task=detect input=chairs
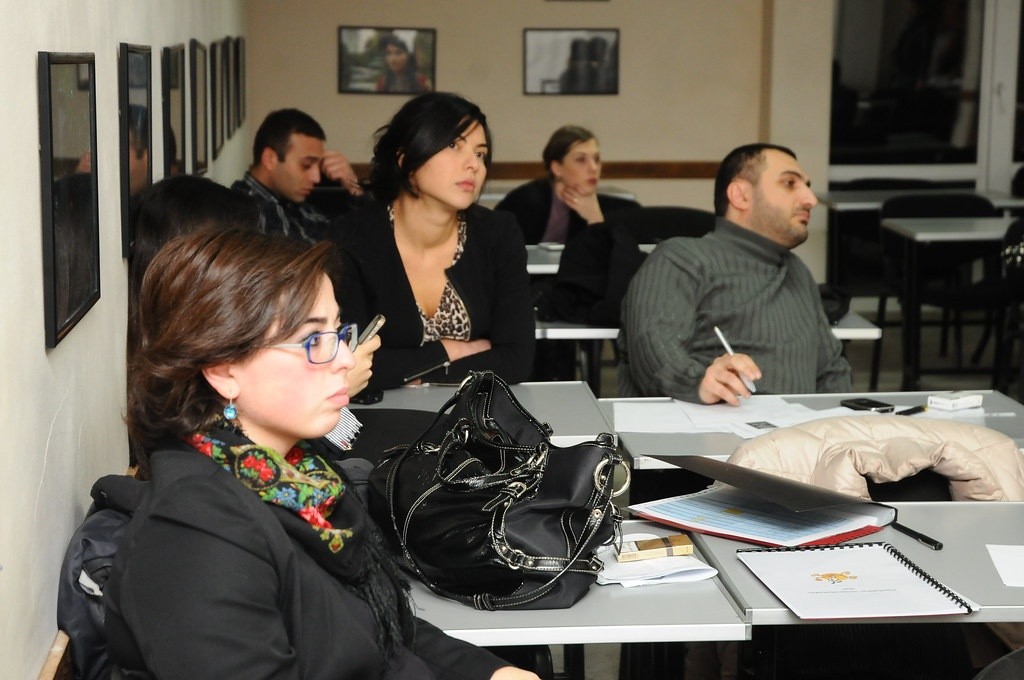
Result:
[868,191,1004,394]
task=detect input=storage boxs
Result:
[926,394,982,411]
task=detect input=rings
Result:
[573,198,577,202]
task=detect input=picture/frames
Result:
[522,28,619,96]
[38,35,247,350]
[337,25,437,95]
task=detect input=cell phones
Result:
[538,242,566,251]
[357,313,386,344]
[840,397,895,414]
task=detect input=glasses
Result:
[254,322,359,363]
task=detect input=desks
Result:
[356,191,1024,646]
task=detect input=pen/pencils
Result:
[890,521,943,552]
[713,325,758,395]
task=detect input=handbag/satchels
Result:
[367,370,631,610]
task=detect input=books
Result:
[740,541,980,619]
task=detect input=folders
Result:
[616,451,899,548]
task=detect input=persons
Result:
[494,124,617,380]
[129,105,177,192]
[230,109,364,242]
[50,172,97,322]
[129,175,250,332]
[106,226,541,680]
[319,91,536,388]
[613,143,853,507]
[375,35,432,92]
